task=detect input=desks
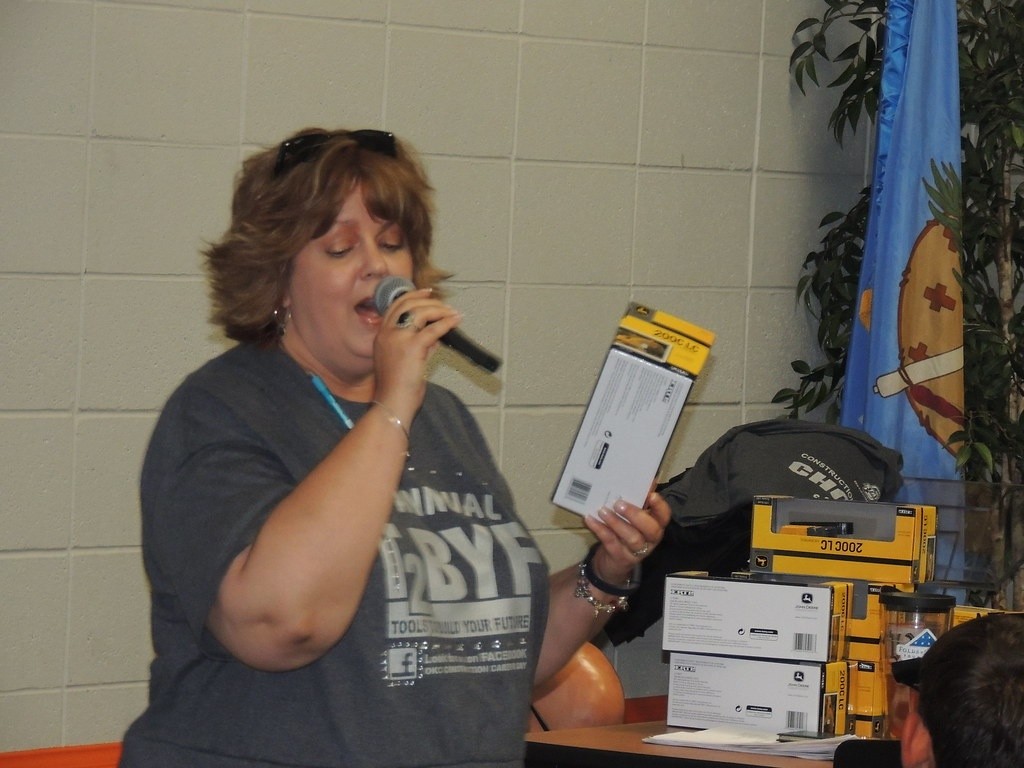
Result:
[524,720,832,766]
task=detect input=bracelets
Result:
[369,400,411,458]
[575,538,643,618]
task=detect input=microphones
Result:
[372,275,501,373]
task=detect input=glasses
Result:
[892,659,926,691]
[277,130,396,177]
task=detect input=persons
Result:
[900,614,1024,768]
[118,124,672,768]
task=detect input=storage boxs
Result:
[550,300,716,527]
[660,492,1024,739]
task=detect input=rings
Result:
[396,309,421,331]
[632,542,648,556]
